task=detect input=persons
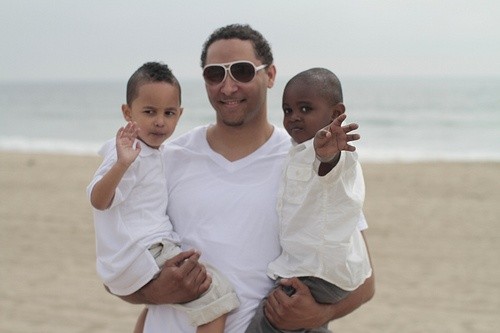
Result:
[89,61,240,331]
[132,23,331,332]
[260,68,369,333]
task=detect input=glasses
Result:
[202,60,267,85]
